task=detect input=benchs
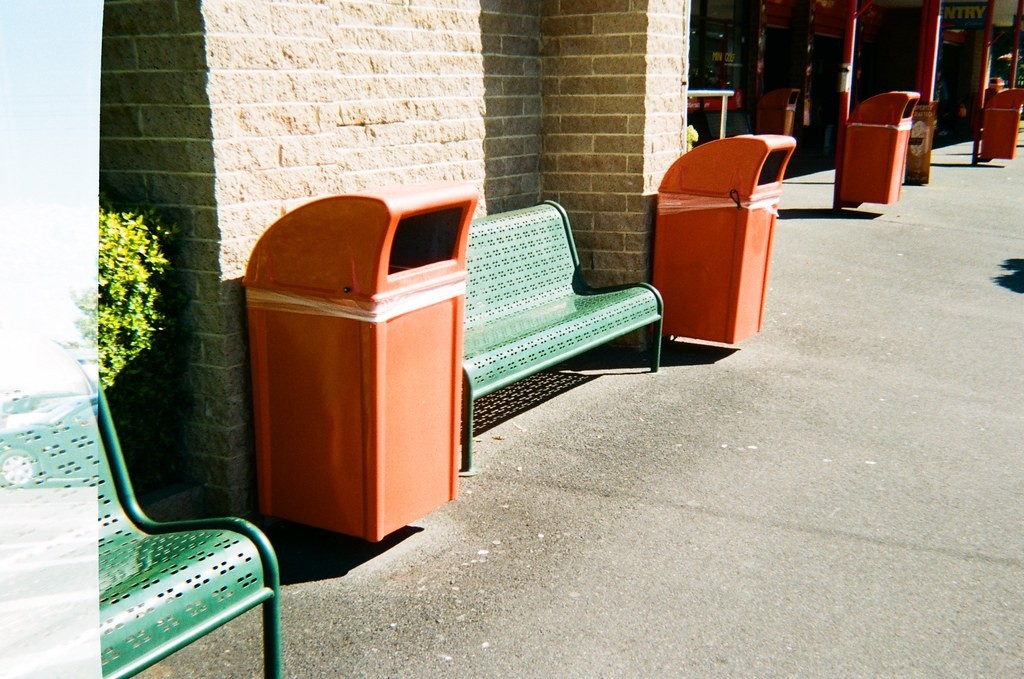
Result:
[0,383,285,679]
[460,199,665,478]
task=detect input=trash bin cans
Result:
[840,91,921,204]
[756,86,801,134]
[242,185,482,542]
[650,134,798,345]
[981,89,1024,160]
